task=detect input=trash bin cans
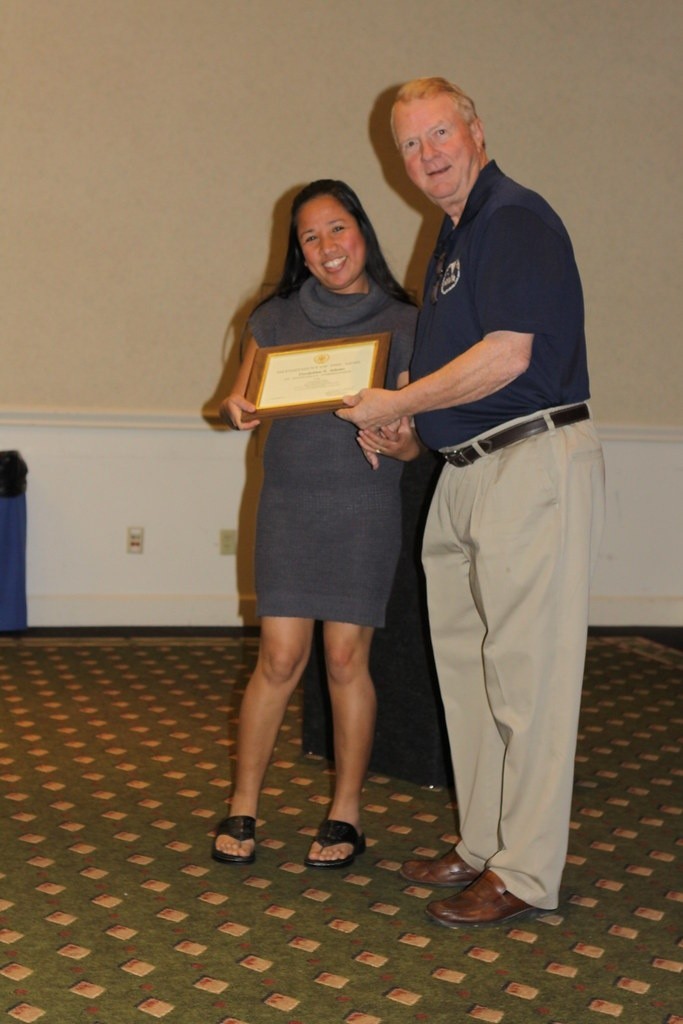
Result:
[0,449,30,634]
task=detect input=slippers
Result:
[304,820,368,868]
[210,817,255,863]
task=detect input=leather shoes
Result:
[399,844,478,887]
[426,864,554,931]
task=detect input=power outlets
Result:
[126,527,145,555]
[220,529,237,556]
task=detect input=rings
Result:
[376,449,381,454]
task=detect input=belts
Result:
[441,403,589,467]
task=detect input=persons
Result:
[209,179,419,870]
[336,78,608,930]
